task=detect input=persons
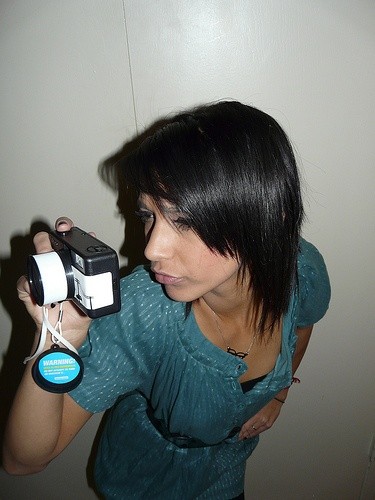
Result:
[2,100,333,499]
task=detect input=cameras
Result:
[28,226,121,319]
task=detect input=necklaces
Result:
[208,305,262,360]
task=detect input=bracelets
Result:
[274,397,286,404]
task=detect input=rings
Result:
[251,425,258,430]
